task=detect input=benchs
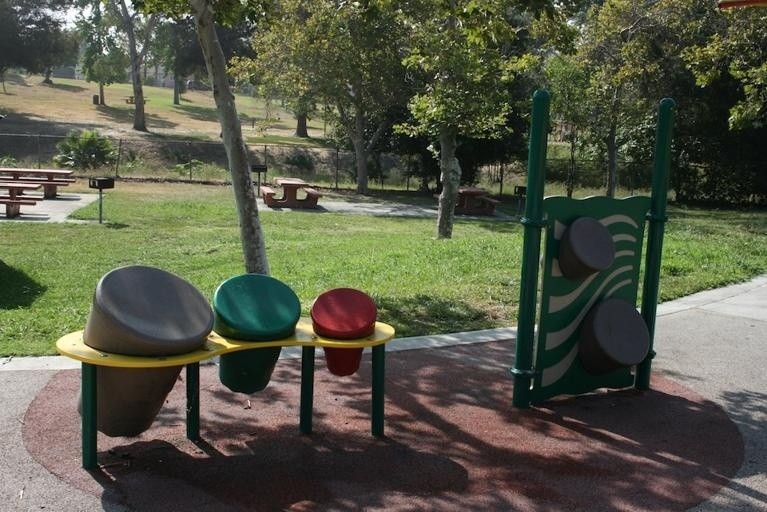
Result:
[477,196,501,216]
[0,176,76,217]
[258,185,276,208]
[302,186,321,208]
[432,192,441,201]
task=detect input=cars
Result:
[185,78,211,92]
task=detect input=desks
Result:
[273,176,308,208]
[454,186,487,215]
[0,168,74,189]
